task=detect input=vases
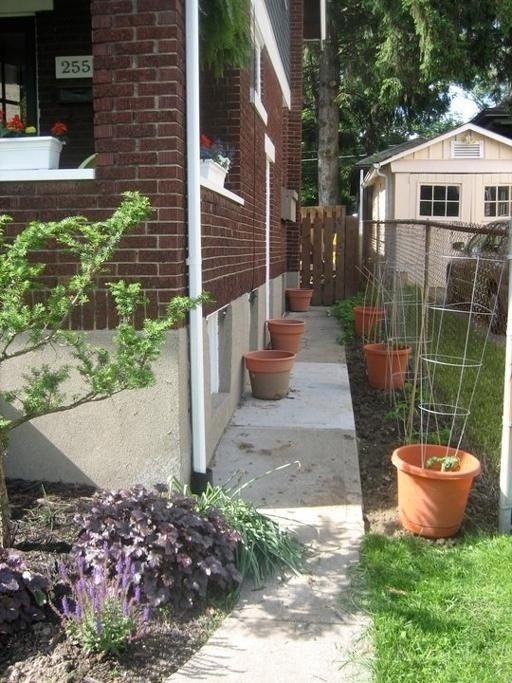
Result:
[0,136,63,169]
[200,159,229,188]
[244,350,296,400]
[268,319,305,354]
[286,288,314,312]
[353,306,387,337]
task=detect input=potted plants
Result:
[363,339,412,389]
[392,443,481,538]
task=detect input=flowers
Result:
[200,134,236,171]
[0,109,69,140]
